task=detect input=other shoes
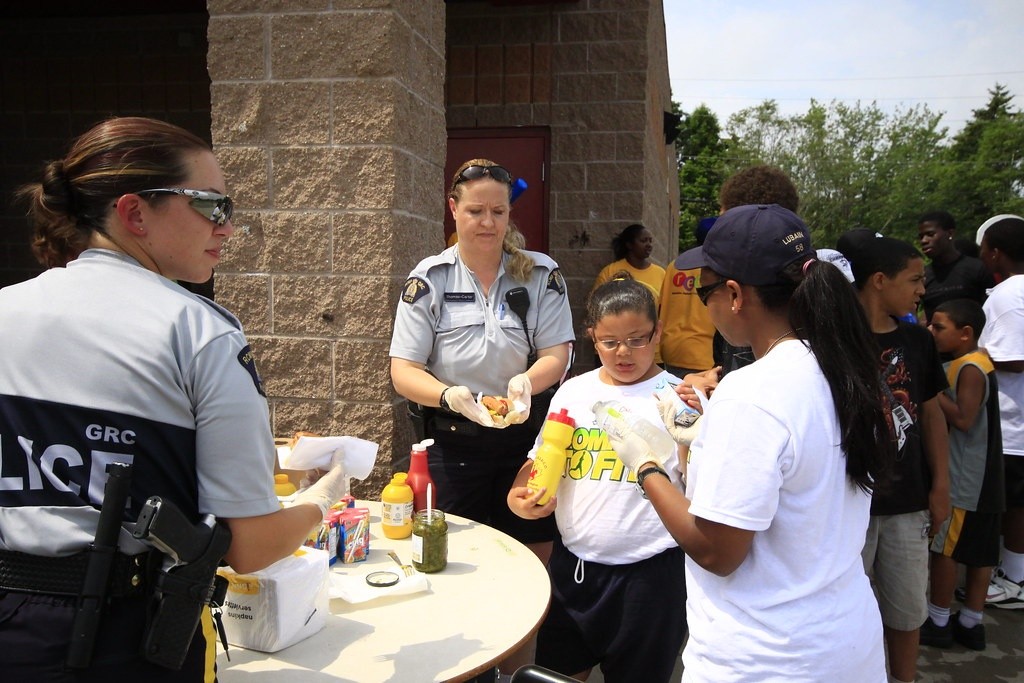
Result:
[919,614,955,649]
[949,610,987,651]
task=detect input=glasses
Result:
[449,164,513,194]
[592,321,657,350]
[695,276,731,308]
[111,187,234,227]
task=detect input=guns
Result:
[130,497,234,670]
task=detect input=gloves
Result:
[607,422,665,479]
[289,447,349,522]
[445,386,508,429]
[656,398,702,446]
[503,373,532,425]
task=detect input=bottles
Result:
[588,397,674,465]
[380,439,437,540]
[524,409,575,506]
[274,474,297,496]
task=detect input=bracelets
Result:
[635,465,671,501]
[439,387,457,415]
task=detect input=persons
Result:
[506,270,707,683]
[687,163,1024,682]
[390,159,575,683]
[608,202,891,683]
[0,118,348,683]
[593,224,667,371]
[661,215,720,380]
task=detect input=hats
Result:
[815,249,855,283]
[673,202,817,286]
[836,227,883,260]
[693,217,717,242]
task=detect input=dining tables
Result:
[210,496,552,683]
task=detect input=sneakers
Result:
[953,558,1024,611]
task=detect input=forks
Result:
[388,552,415,578]
